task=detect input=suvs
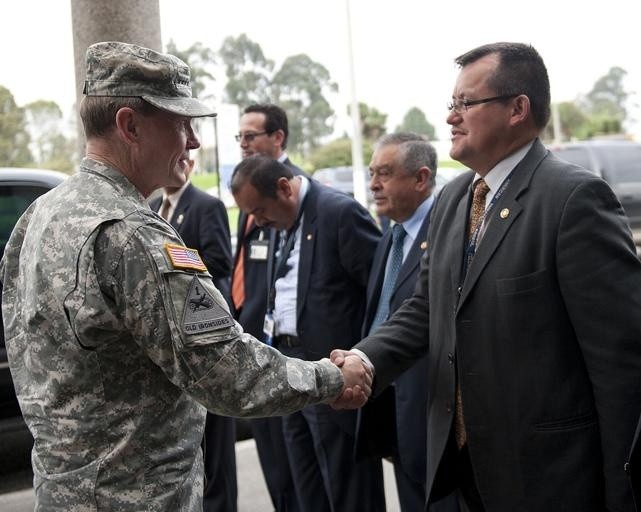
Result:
[544,139,641,248]
[312,165,372,205]
[0,168,251,458]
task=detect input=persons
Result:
[331,41,641,512]
[0,39,372,512]
[228,154,383,512]
[348,132,444,512]
[374,213,389,233]
[228,103,310,511]
[145,152,237,512]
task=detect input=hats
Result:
[84,41,218,117]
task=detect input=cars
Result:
[434,168,459,196]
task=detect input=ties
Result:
[231,214,255,309]
[161,199,170,220]
[368,224,407,336]
[465,178,491,270]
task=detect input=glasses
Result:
[235,131,269,141]
[447,94,517,113]
